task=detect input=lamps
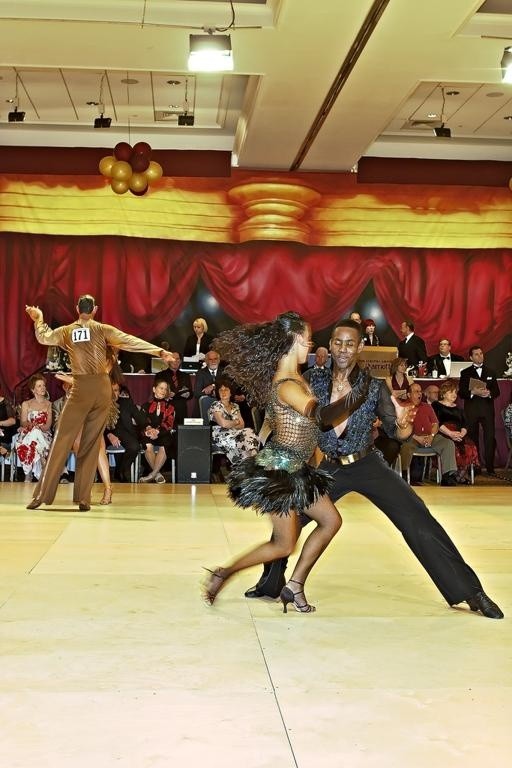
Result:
[186,26,234,74]
[432,124,452,138]
[94,117,112,128]
[501,46,512,84]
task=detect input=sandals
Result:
[24,471,167,484]
[100,487,112,505]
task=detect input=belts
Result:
[323,443,373,466]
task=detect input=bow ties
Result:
[442,356,450,360]
[476,365,482,369]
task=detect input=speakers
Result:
[176,425,211,484]
[433,127,451,136]
[94,117,113,127]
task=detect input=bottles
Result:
[431,359,439,378]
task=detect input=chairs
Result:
[0,454,7,482]
[501,409,512,473]
[104,450,135,484]
[390,453,402,478]
[251,406,263,436]
[199,395,229,483]
[406,448,443,487]
[134,402,176,484]
[428,453,476,485]
[14,400,25,482]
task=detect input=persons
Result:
[245,317,504,619]
[203,311,372,614]
[1,313,511,486]
[24,294,176,511]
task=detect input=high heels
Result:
[245,585,267,597]
[202,566,225,608]
[280,580,316,613]
[466,589,504,618]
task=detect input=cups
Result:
[418,364,426,379]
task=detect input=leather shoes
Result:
[78,500,90,510]
[26,498,52,508]
[442,468,497,486]
